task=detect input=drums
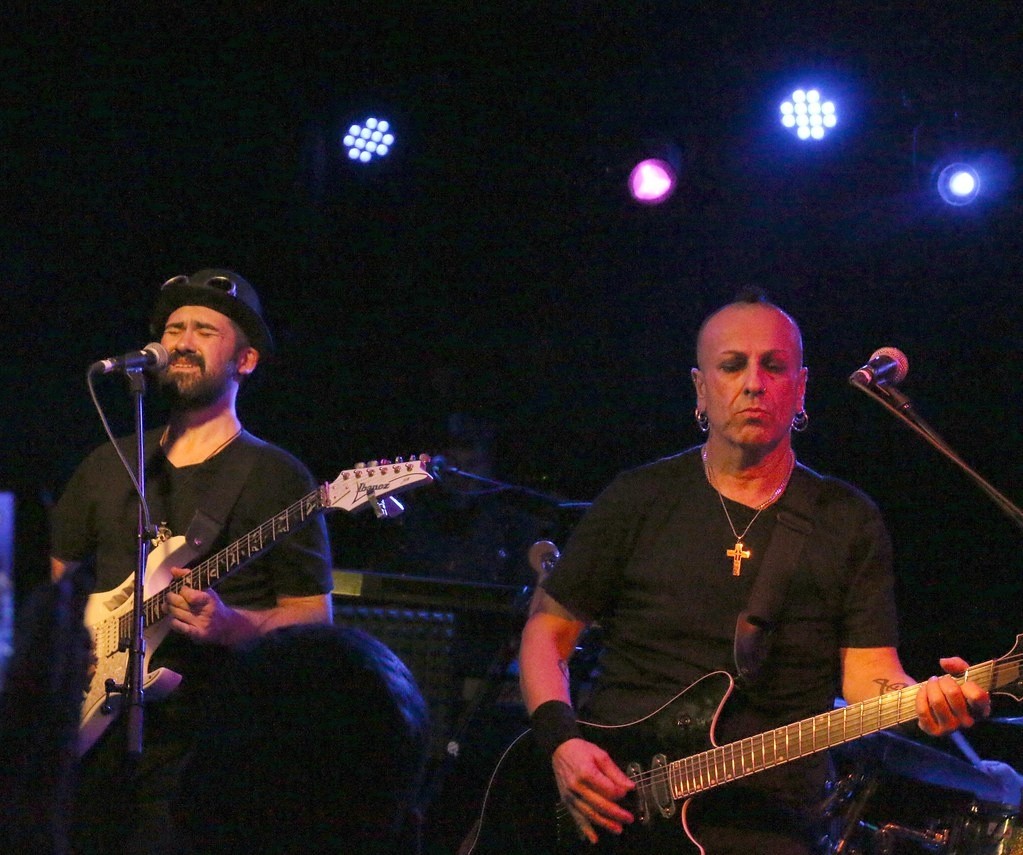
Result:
[924,799,1023,855]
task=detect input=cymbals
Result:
[989,716,1023,726]
[823,710,1004,797]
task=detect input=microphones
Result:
[529,541,560,574]
[848,346,908,387]
[90,342,168,375]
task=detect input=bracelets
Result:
[530,701,585,764]
[902,719,922,735]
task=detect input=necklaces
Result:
[162,428,241,462]
[706,448,794,576]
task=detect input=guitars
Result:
[464,634,1023,855]
[41,451,438,763]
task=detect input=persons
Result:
[518,299,992,855]
[182,623,426,855]
[49,268,333,855]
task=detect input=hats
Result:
[148,266,279,355]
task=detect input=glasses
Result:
[158,273,238,297]
